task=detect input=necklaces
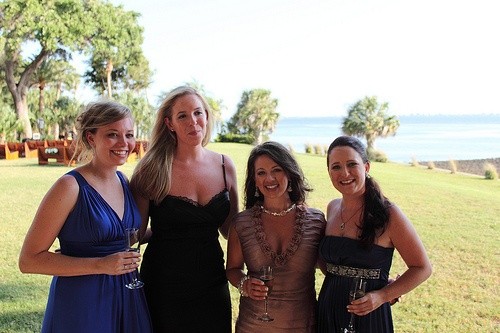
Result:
[261,203,297,216]
[340,203,364,230]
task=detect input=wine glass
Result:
[125,227,146,290]
[256,265,274,322]
[340,277,367,333]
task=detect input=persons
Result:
[313,135,433,333]
[18,100,153,333]
[226,141,398,333]
[128,83,239,333]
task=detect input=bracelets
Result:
[237,275,252,297]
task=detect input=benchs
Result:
[0,138,149,168]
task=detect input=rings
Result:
[123,265,126,269]
